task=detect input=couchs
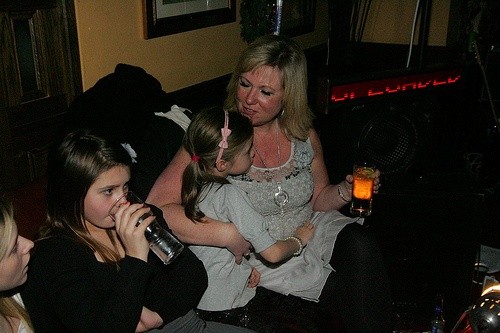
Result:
[1,160,391,333]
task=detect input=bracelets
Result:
[285,236,303,256]
[338,183,351,202]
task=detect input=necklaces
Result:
[252,118,290,207]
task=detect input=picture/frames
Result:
[141,0,236,40]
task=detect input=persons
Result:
[18,131,260,333]
[0,196,33,333]
[181,109,313,333]
[146,33,394,332]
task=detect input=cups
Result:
[108,192,184,264]
[350,162,377,216]
[473,264,488,284]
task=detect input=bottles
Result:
[428,306,445,333]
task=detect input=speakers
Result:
[353,102,435,200]
